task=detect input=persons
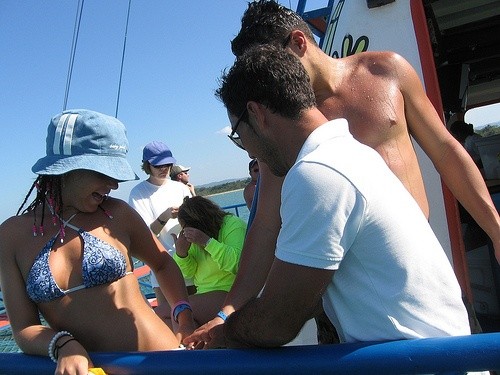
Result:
[129,0,500,349]
[0,110,192,375]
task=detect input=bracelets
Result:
[157,218,167,225]
[49,330,76,364]
[174,304,190,322]
[201,238,210,248]
[171,300,191,323]
[216,310,227,323]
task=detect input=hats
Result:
[143,141,176,166]
[31,109,141,180]
[171,165,190,179]
[249,159,256,171]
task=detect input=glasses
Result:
[228,108,247,150]
[251,169,259,172]
